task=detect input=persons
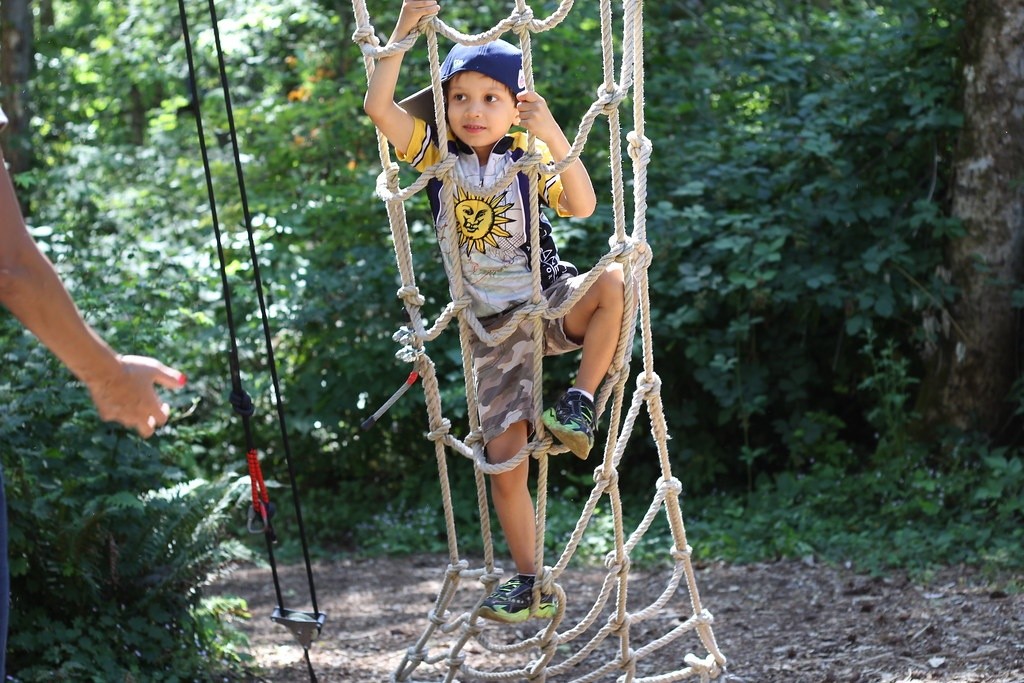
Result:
[0,105,187,437]
[363,0,638,624]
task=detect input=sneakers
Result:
[477,575,558,623]
[542,391,598,460]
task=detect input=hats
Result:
[399,39,525,138]
